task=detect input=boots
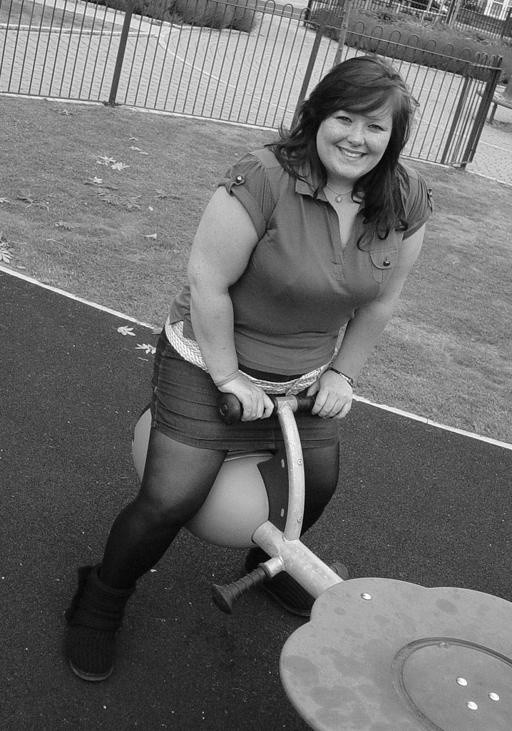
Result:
[67,564,137,681]
[247,549,315,617]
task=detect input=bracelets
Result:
[329,368,354,387]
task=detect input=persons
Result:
[63,56,436,683]
